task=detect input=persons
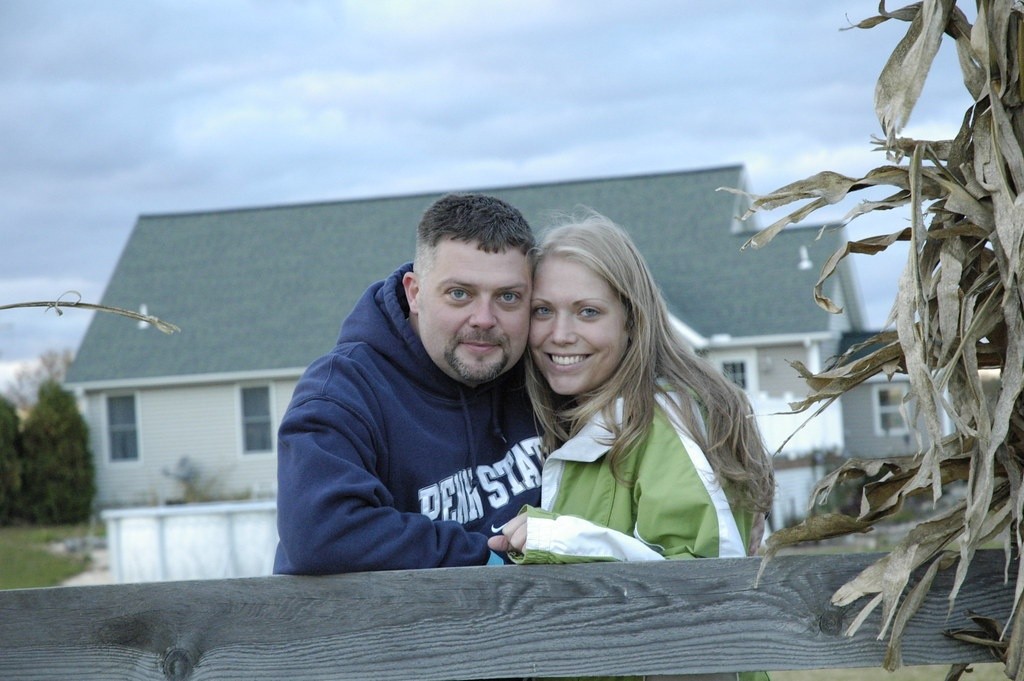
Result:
[273,194,548,577]
[487,209,776,566]
